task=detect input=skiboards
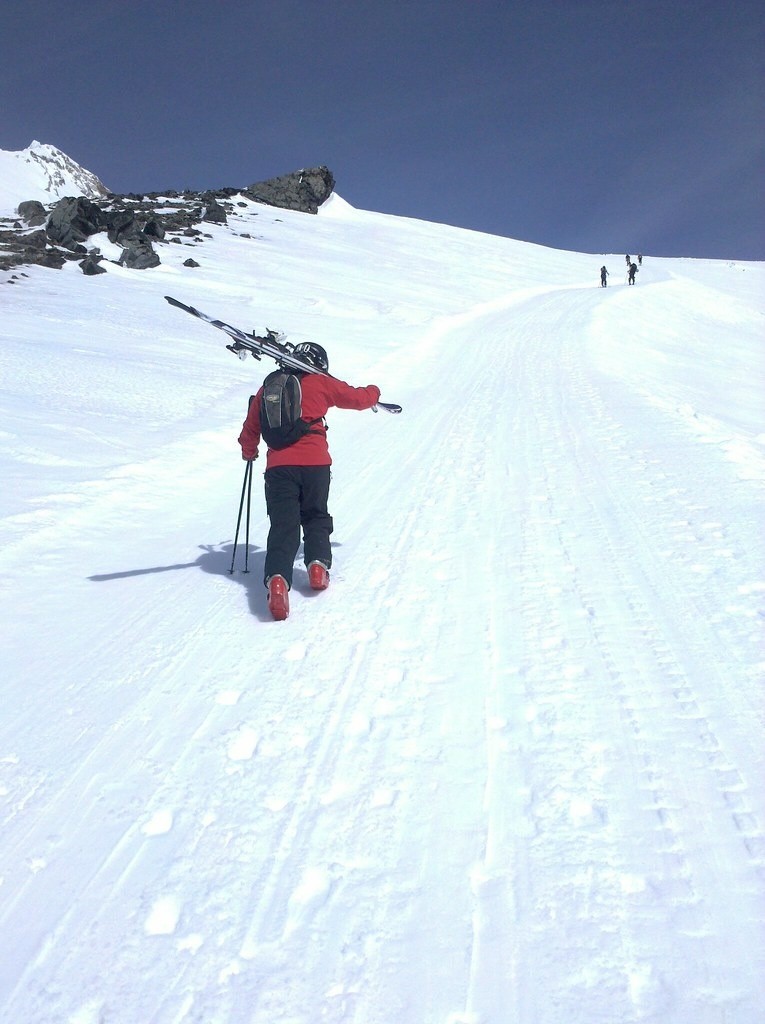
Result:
[166,295,405,415]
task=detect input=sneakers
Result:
[266,574,289,621]
[306,560,329,590]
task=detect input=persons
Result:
[239,342,380,622]
[600,253,643,287]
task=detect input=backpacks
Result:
[260,369,322,450]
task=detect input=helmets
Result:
[292,342,328,374]
